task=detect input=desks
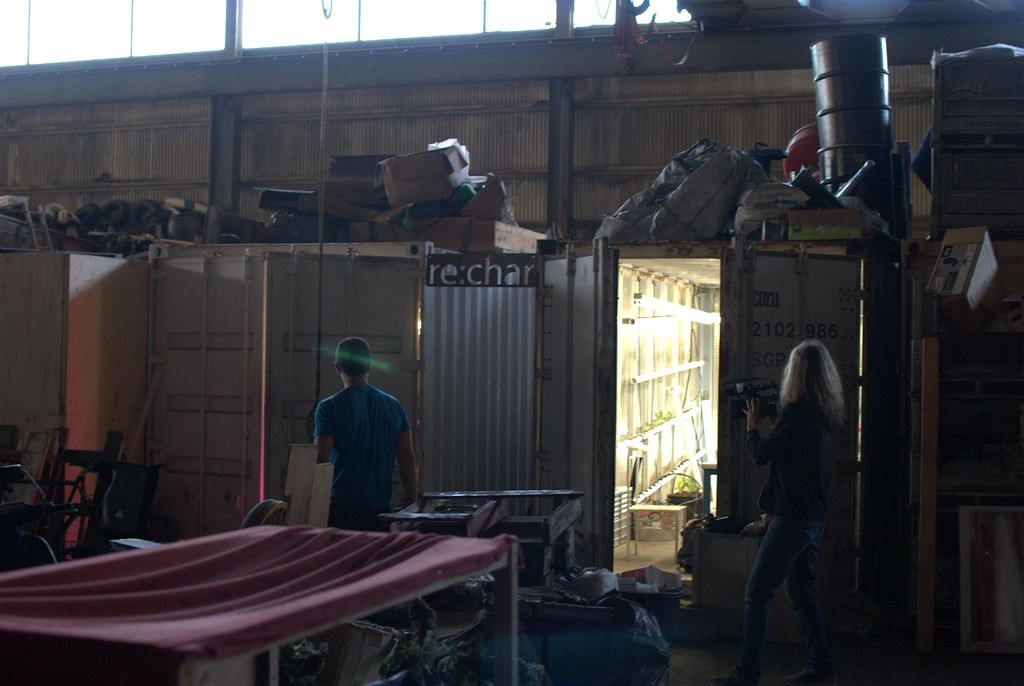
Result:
[626,504,687,561]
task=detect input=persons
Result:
[704,339,846,686]
[313,337,416,533]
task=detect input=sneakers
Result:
[706,672,759,686]
[783,664,838,686]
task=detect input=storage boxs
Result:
[255,137,547,254]
[921,226,1007,336]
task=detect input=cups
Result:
[622,565,656,584]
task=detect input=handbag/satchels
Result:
[756,479,775,512]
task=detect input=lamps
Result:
[631,359,705,385]
[620,292,721,331]
